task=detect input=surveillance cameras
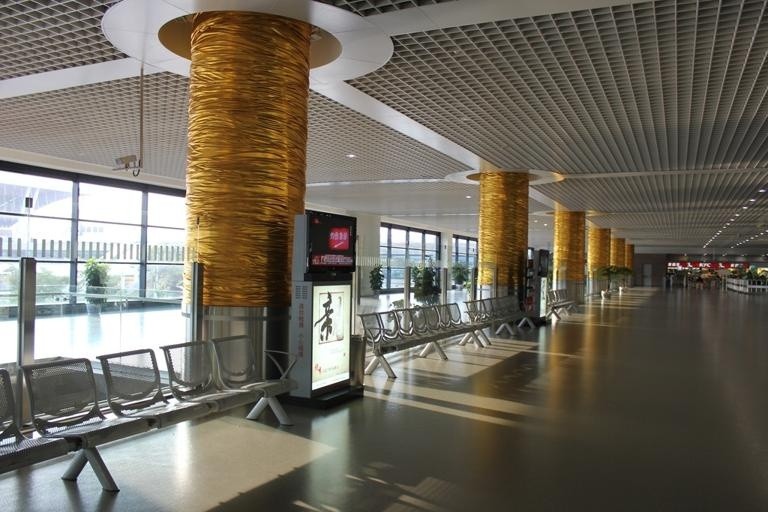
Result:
[115,155,137,166]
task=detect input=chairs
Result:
[355,287,580,379]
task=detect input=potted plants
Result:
[369,264,385,298]
[451,263,471,291]
[596,265,633,300]
[83,255,112,315]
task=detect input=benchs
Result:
[1,337,298,491]
